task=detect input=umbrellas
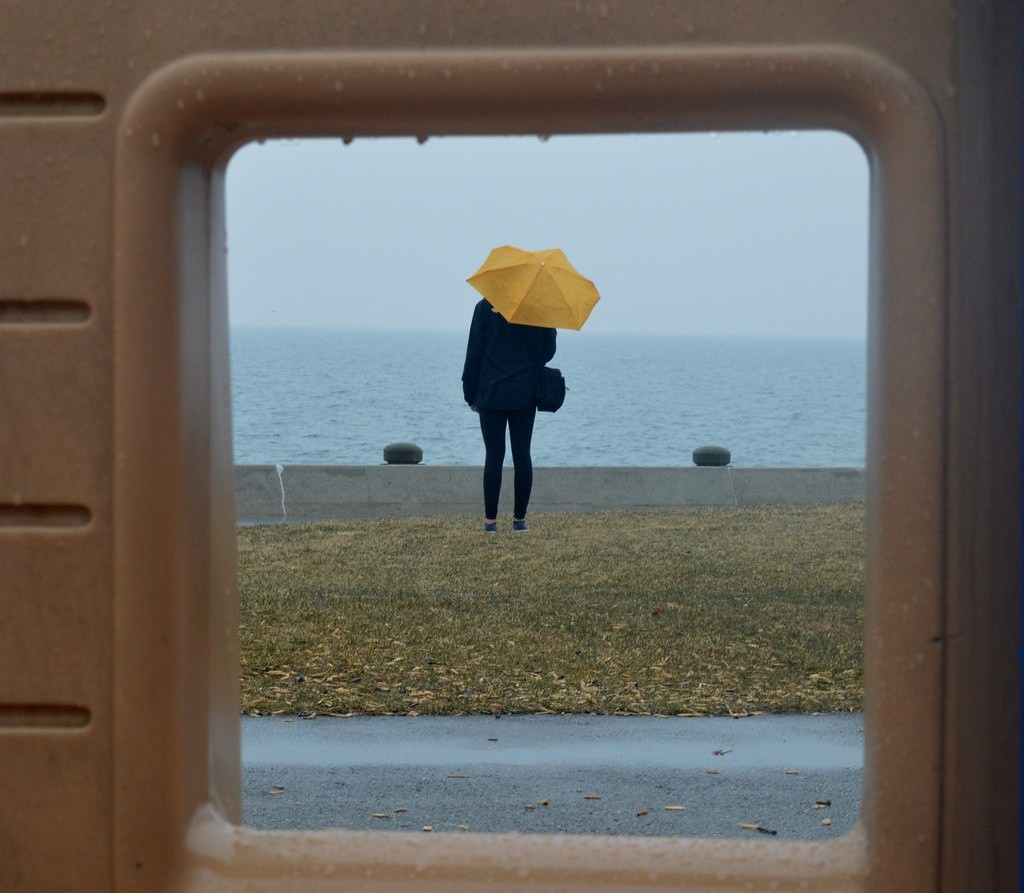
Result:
[466,245,600,330]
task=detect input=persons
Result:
[462,297,557,533]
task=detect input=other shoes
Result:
[485,524,497,533]
[513,521,529,531]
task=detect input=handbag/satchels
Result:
[525,367,567,413]
